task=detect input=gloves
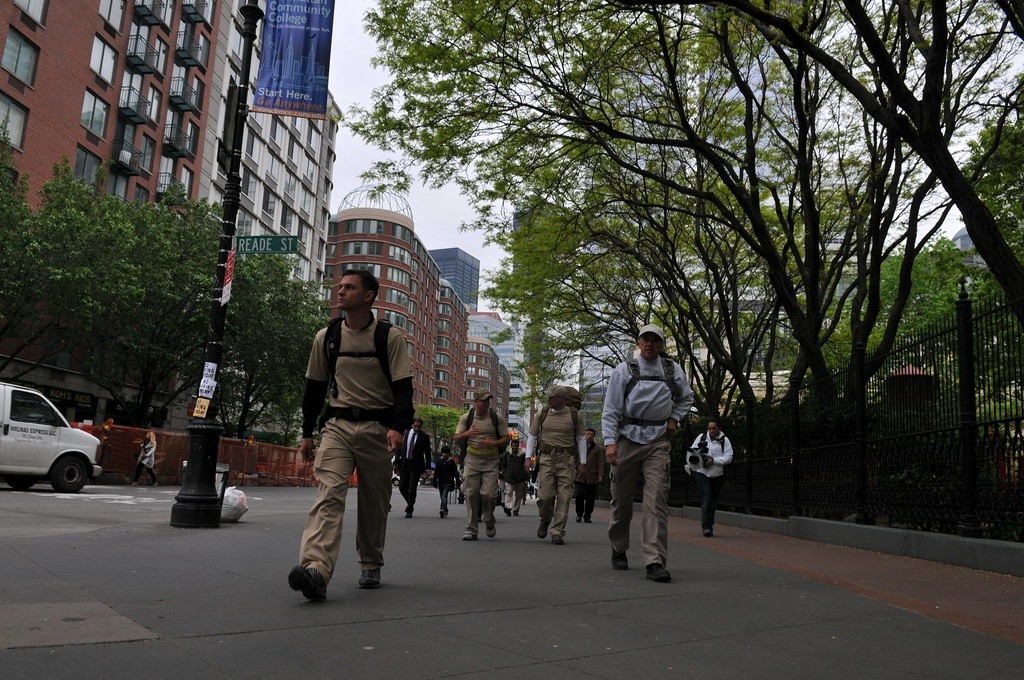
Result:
[704,457,714,469]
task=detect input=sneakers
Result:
[358,568,381,589]
[288,565,327,603]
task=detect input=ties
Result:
[408,433,416,460]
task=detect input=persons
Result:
[453,439,540,518]
[131,431,158,487]
[452,388,508,541]
[287,270,416,603]
[394,417,431,518]
[575,428,604,524]
[523,385,588,545]
[600,324,694,582]
[690,419,734,537]
[433,446,459,518]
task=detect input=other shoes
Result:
[405,505,413,512]
[703,526,713,537]
[612,547,628,570]
[130,482,138,487]
[405,513,412,518]
[152,481,158,488]
[440,500,597,523]
[552,535,564,545]
[486,517,496,537]
[463,533,478,541]
[537,520,550,539]
[646,563,671,582]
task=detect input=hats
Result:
[544,385,567,397]
[638,324,664,343]
[474,388,494,400]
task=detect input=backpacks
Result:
[563,387,581,411]
[460,408,506,454]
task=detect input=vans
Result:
[0,382,104,493]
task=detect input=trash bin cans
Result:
[181,462,230,509]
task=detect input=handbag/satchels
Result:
[393,458,401,477]
